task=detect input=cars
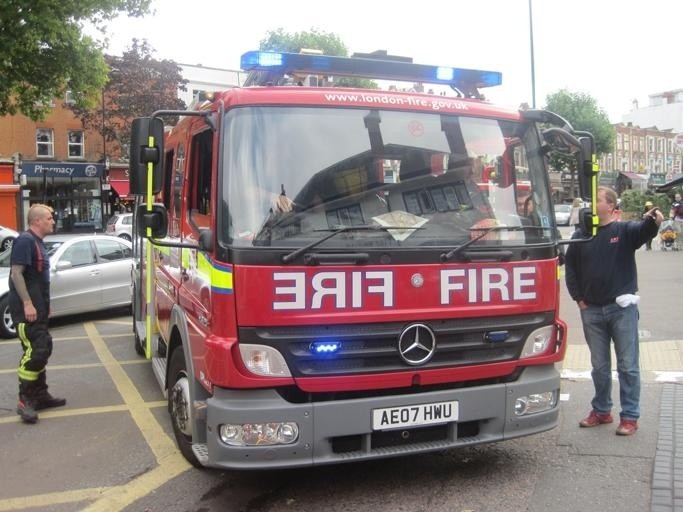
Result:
[553,202,573,226]
[105,210,133,242]
[0,226,132,338]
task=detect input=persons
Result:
[242,175,327,228]
[516,191,565,266]
[7,200,67,425]
[642,201,654,252]
[668,192,683,242]
[659,225,677,250]
[564,185,663,437]
[570,196,581,230]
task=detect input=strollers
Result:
[659,224,678,251]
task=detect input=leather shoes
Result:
[645,245,652,250]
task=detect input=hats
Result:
[644,201,654,207]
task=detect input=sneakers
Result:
[614,417,638,436]
[17,384,38,422]
[33,389,66,410]
[579,409,612,428]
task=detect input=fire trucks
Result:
[129,49,600,473]
